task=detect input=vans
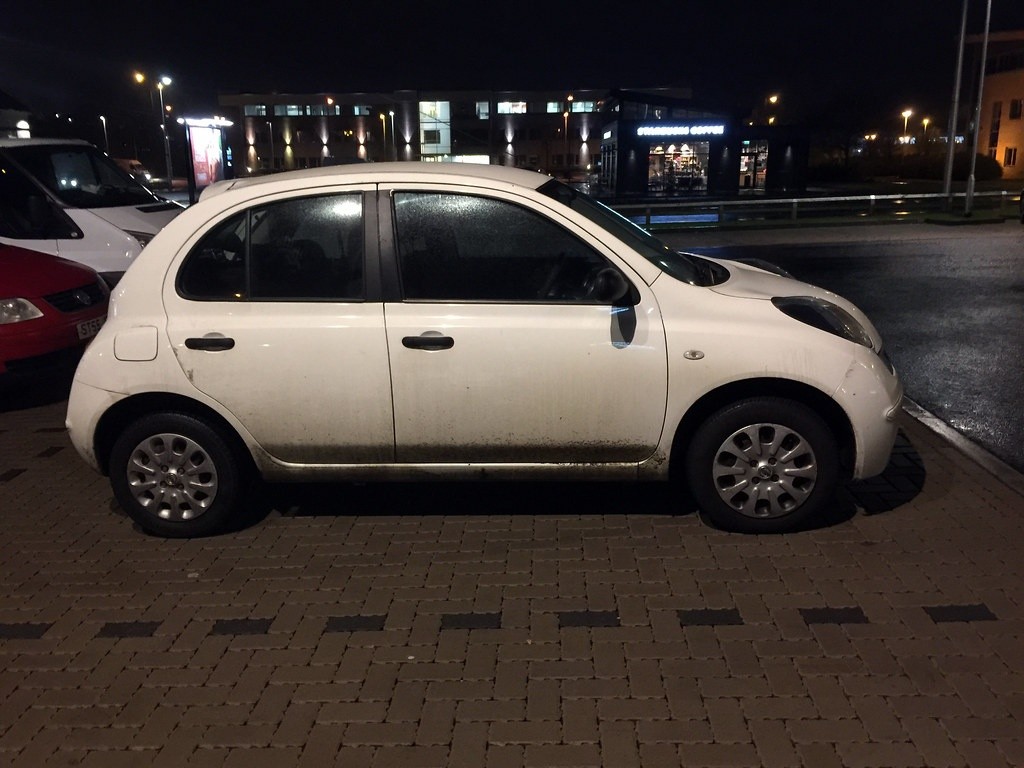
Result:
[0,138,187,290]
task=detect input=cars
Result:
[1,243,110,377]
[63,161,907,534]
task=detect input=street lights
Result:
[159,77,172,192]
[923,120,928,130]
[903,111,910,143]
